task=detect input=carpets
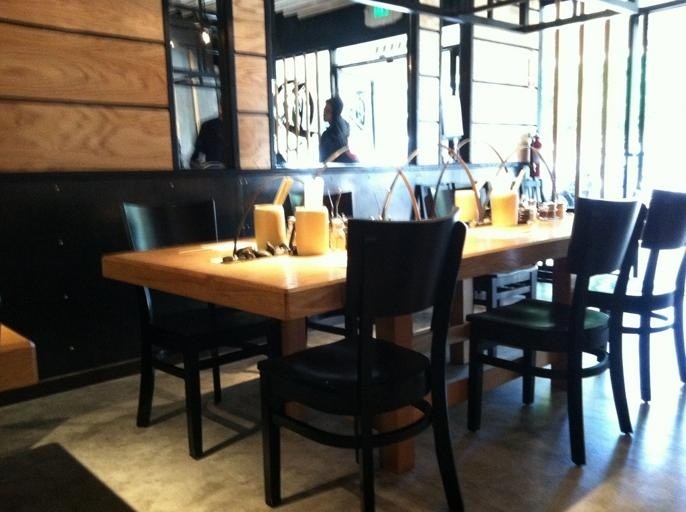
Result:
[1,442,135,510]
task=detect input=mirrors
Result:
[265,1,417,170]
[162,0,239,171]
[439,18,470,166]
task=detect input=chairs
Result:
[286,191,355,217]
[260,216,466,511]
[467,196,647,467]
[520,180,553,279]
[122,200,273,459]
[419,182,538,360]
[585,189,685,401]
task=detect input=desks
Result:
[0,323,40,391]
[103,214,574,510]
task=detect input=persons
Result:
[185,113,285,172]
[312,94,359,163]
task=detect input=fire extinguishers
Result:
[531,135,541,176]
